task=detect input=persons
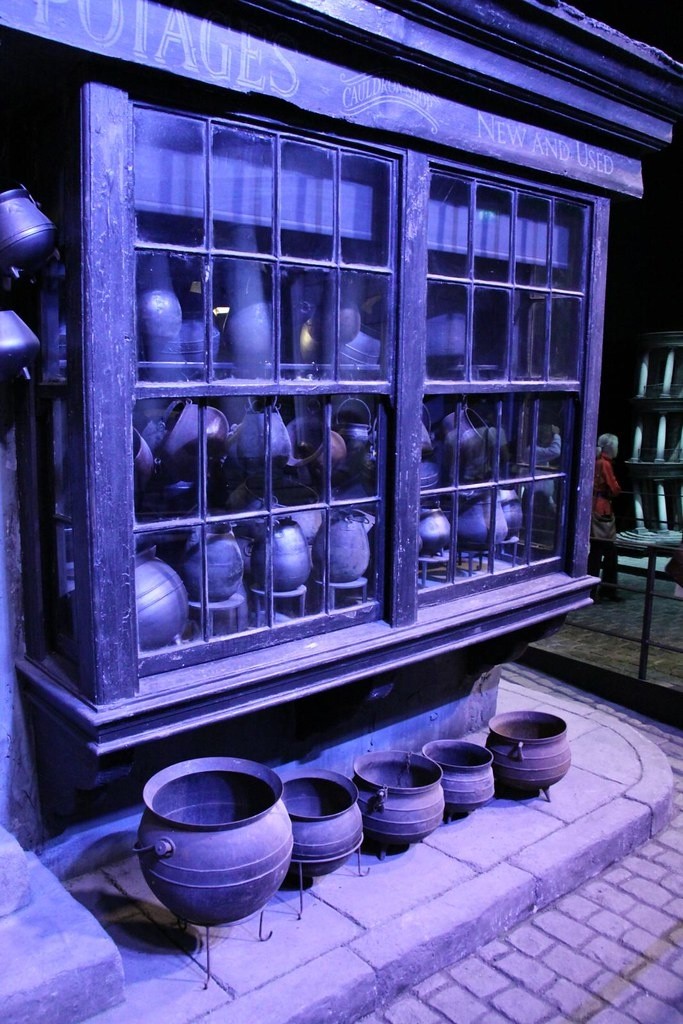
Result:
[588,433,624,600]
[522,423,561,555]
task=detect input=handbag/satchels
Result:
[590,511,616,542]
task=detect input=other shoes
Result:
[598,590,625,602]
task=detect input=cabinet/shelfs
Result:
[14,55,612,757]
[613,328,683,558]
